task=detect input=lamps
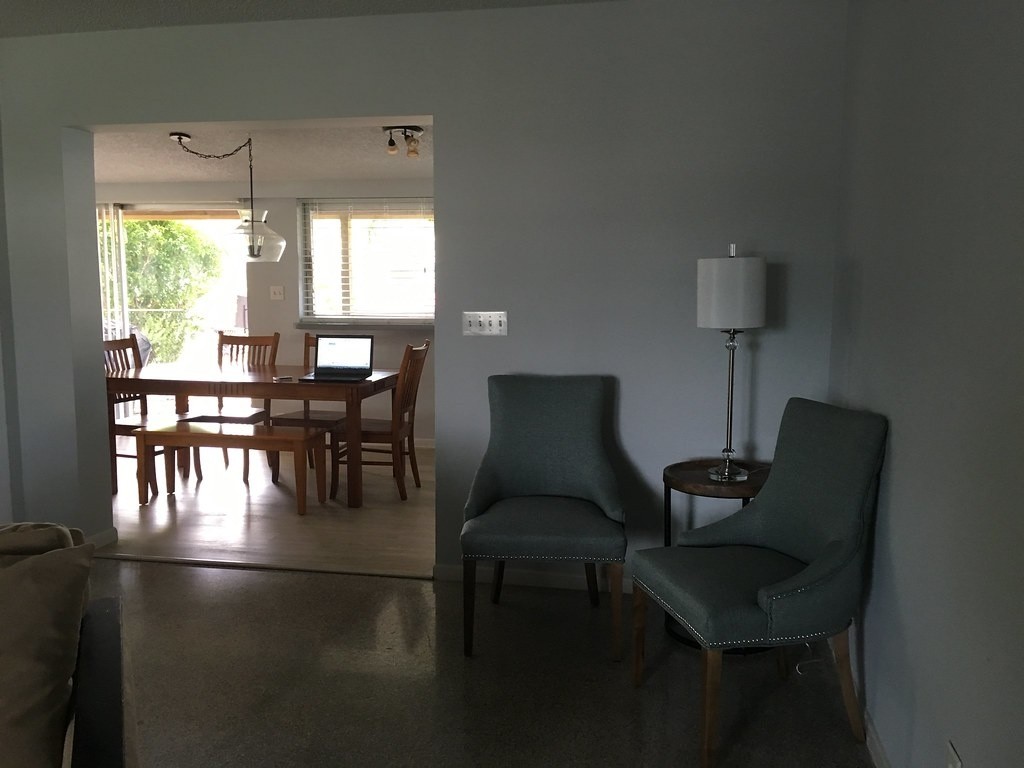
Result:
[382,125,424,157]
[696,243,766,482]
[170,132,286,262]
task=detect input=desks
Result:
[106,365,405,508]
[664,460,775,655]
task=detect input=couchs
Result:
[0,522,139,768]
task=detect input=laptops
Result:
[297,334,374,382]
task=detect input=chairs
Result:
[193,331,280,481]
[269,333,349,483]
[102,334,177,495]
[459,374,626,663]
[631,397,888,768]
[330,339,431,501]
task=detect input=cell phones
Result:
[273,376,293,381]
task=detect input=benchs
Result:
[130,421,327,516]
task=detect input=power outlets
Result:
[462,311,508,335]
